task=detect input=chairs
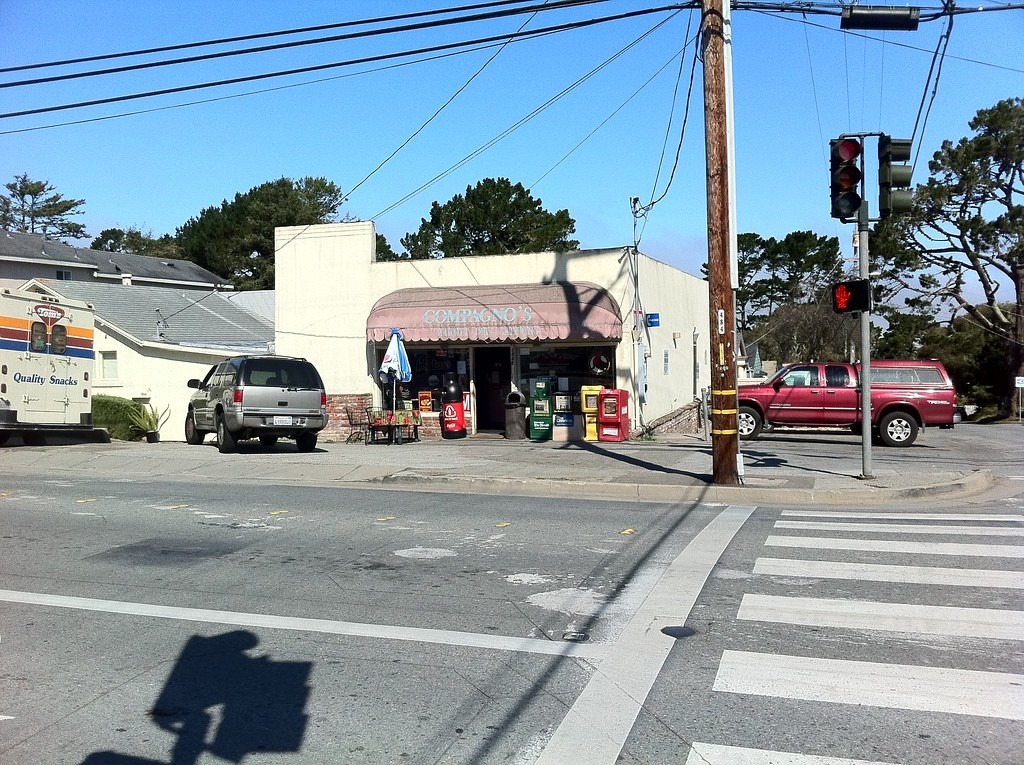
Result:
[343,406,392,446]
[793,375,805,386]
[266,377,283,387]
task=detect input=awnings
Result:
[366,281,623,342]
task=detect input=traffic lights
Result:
[830,277,871,314]
[879,134,913,218]
[829,138,862,218]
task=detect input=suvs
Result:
[185,353,329,453]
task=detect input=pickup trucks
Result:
[738,357,959,448]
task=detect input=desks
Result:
[371,410,423,445]
[411,399,435,410]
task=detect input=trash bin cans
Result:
[440,381,467,439]
[505,391,526,439]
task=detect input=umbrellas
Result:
[379,330,412,413]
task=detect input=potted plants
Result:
[127,403,171,443]
